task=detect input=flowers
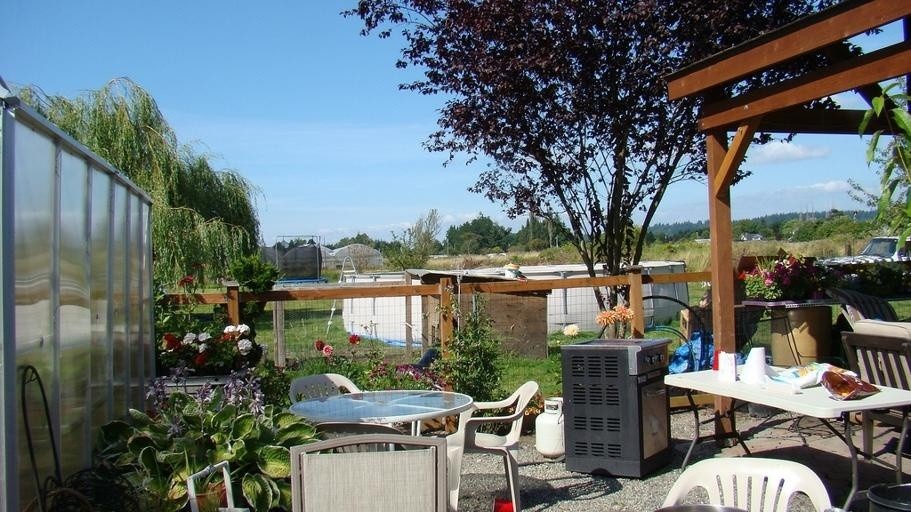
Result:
[99,359,333,512]
[303,334,362,379]
[595,305,620,338]
[158,275,254,376]
[563,325,580,344]
[737,254,821,301]
[612,305,634,338]
[365,363,450,393]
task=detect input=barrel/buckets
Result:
[866,482,911,512]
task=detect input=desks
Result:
[290,389,474,438]
[663,364,911,511]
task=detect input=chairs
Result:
[290,434,447,512]
[446,381,539,512]
[663,457,831,512]
[841,319,911,460]
[290,373,394,452]
[825,287,899,331]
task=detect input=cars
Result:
[819,234,907,281]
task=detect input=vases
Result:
[196,482,227,512]
